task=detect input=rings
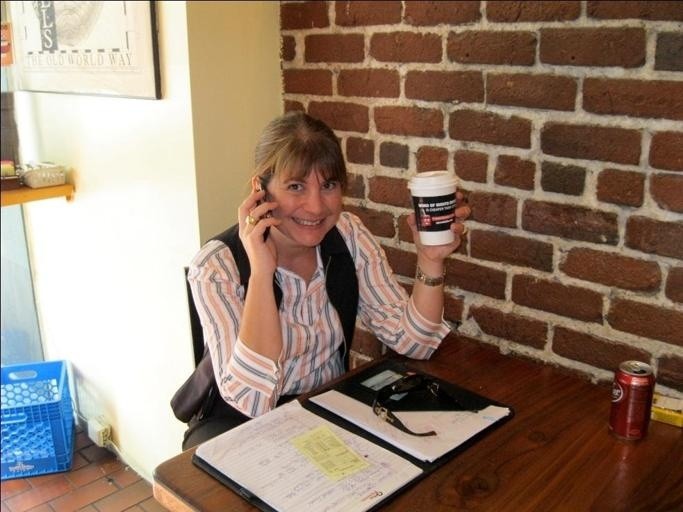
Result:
[243,214,256,224]
[461,222,468,237]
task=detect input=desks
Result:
[153,334,682,511]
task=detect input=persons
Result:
[169,109,471,457]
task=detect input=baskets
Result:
[0,359,75,482]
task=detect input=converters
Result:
[88,418,111,447]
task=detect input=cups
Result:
[409,169,459,245]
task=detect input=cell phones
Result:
[256,181,271,242]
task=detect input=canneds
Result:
[607,360,656,441]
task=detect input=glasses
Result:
[374,373,478,437]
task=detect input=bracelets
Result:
[414,261,448,287]
